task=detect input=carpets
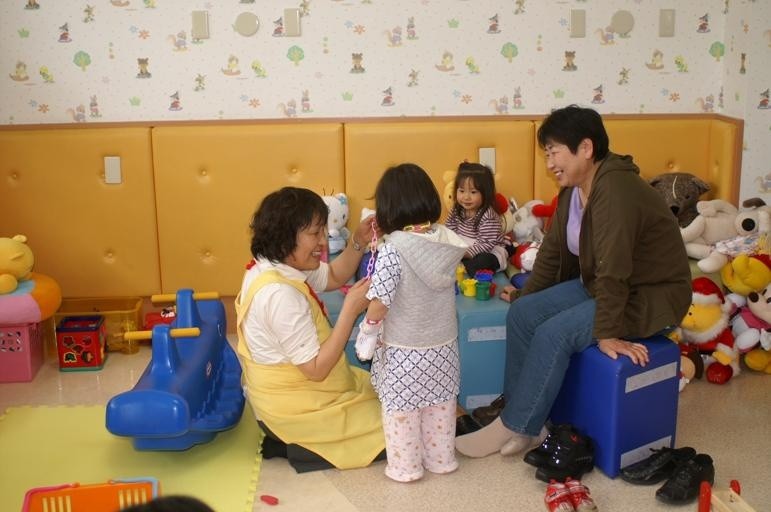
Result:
[254,421,757,510]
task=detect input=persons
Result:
[236,188,388,475]
[452,105,693,460]
[355,162,473,482]
[443,164,508,278]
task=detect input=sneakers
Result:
[524,424,599,511]
[455,394,503,435]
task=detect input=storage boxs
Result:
[54,295,146,356]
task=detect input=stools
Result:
[567,331,678,477]
[456,259,521,407]
[319,277,369,377]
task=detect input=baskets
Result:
[21,478,160,512]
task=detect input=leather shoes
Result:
[622,446,714,502]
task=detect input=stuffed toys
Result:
[0,235,35,293]
[509,171,771,384]
[320,194,350,255]
